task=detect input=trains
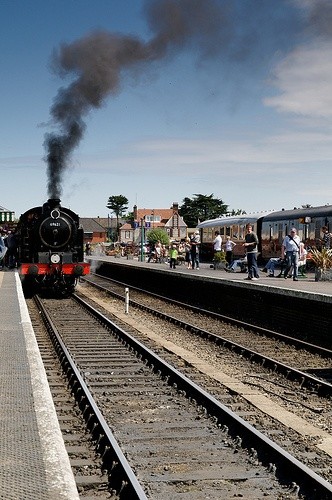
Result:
[196,205,332,269]
[14,198,91,300]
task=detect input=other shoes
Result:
[244,276,251,280]
[260,269,267,273]
[285,276,287,279]
[269,274,274,277]
[287,275,291,278]
[251,277,258,281]
[293,277,298,281]
[275,275,281,278]
[224,268,231,273]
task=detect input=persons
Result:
[0,224,18,270]
[225,236,236,266]
[85,237,307,277]
[212,231,222,252]
[282,227,301,282]
[321,226,332,249]
[194,231,200,242]
[242,223,260,280]
